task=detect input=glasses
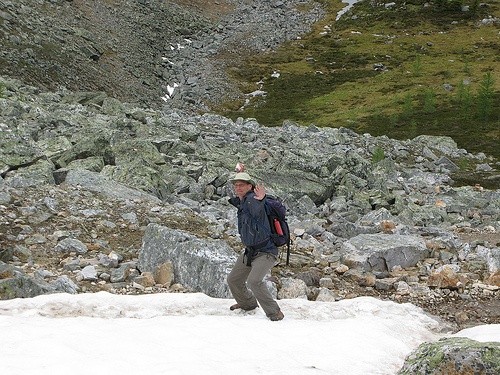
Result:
[234,183,247,188]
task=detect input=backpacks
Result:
[236,192,290,247]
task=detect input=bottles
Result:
[274,218,284,236]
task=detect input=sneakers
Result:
[230,303,259,310]
[267,310,284,321]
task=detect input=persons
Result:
[227,172,284,321]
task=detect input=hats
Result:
[229,173,256,189]
